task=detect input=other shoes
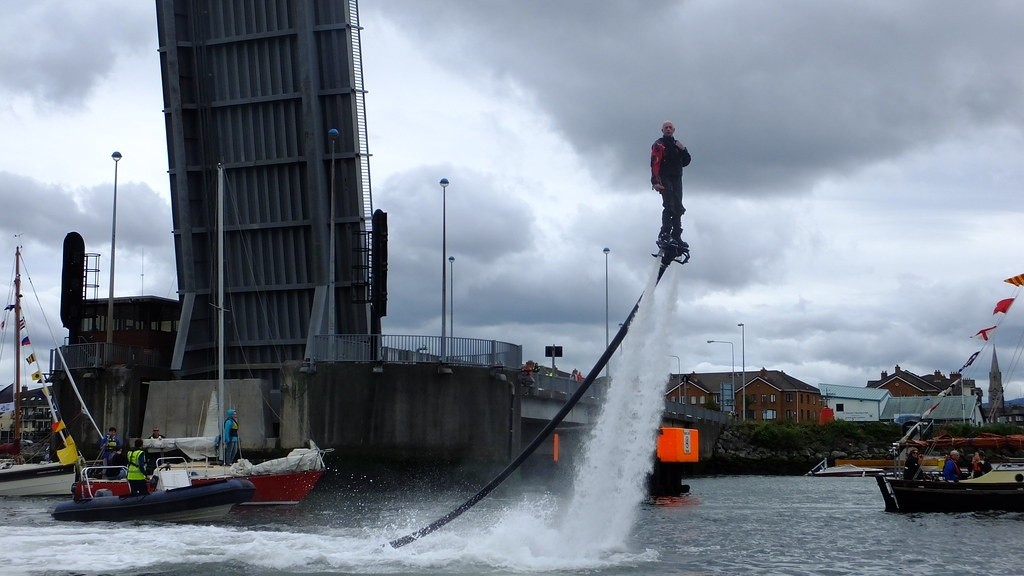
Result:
[671,233,689,248]
[658,236,678,247]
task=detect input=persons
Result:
[650,120,691,248]
[943,450,992,480]
[224,409,240,467]
[520,359,586,402]
[904,448,931,481]
[100,426,166,496]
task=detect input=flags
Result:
[897,273,1024,444]
[0,304,80,465]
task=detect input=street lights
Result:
[102,150,125,365]
[706,339,735,412]
[738,322,746,423]
[603,247,612,388]
[439,176,449,361]
[447,256,457,361]
[663,353,681,404]
[328,127,340,360]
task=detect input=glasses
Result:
[913,452,918,454]
[153,430,159,432]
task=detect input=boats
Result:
[805,267,1024,515]
[0,243,327,523]
